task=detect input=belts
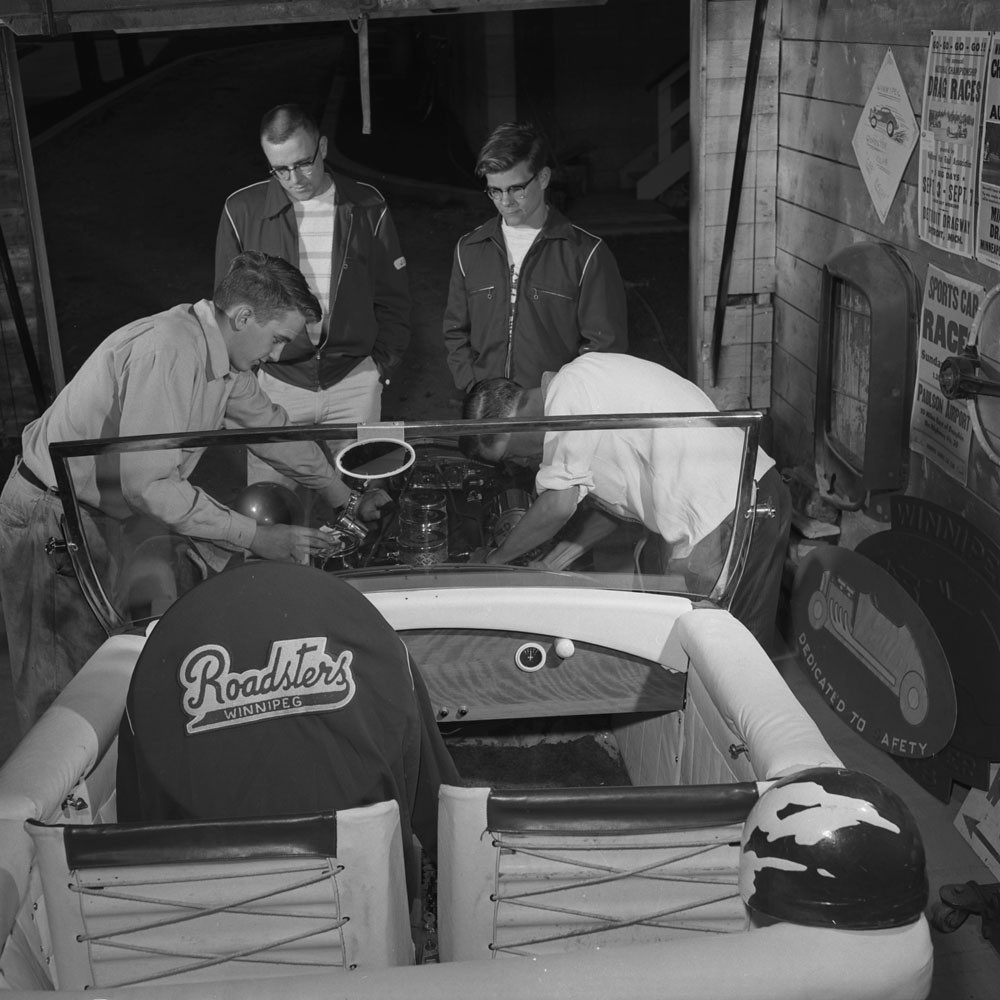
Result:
[16,459,60,498]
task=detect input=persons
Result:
[459,350,793,649]
[440,121,635,571]
[0,248,391,747]
[214,104,412,493]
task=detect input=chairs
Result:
[439,782,759,959]
[23,799,418,993]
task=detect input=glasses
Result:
[270,129,322,182]
[482,173,536,201]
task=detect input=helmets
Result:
[740,764,928,929]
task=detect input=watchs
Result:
[348,490,361,511]
